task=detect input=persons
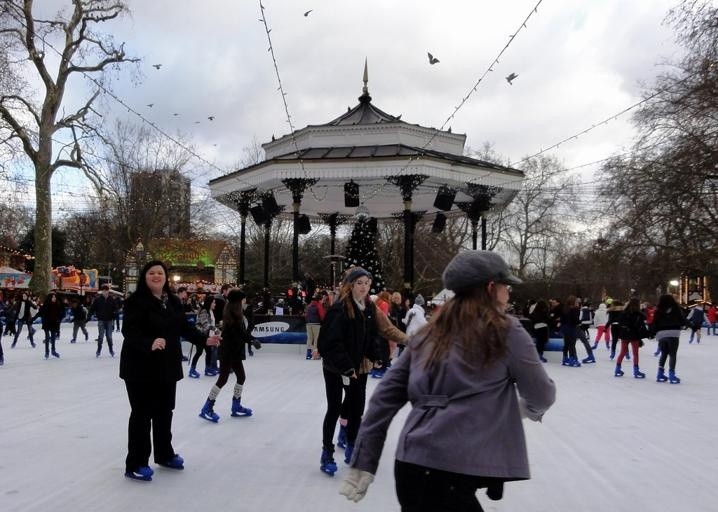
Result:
[175,284,262,424]
[0,285,122,366]
[304,267,427,477]
[118,260,224,481]
[338,249,556,511]
[521,293,593,368]
[590,294,717,384]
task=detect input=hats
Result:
[227,290,247,303]
[444,249,523,292]
[346,267,368,281]
[415,294,424,305]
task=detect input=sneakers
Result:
[189,368,199,376]
[563,356,569,363]
[167,457,183,465]
[615,364,623,374]
[231,398,252,413]
[306,349,312,357]
[136,466,153,476]
[345,445,354,458]
[338,430,347,445]
[202,399,219,420]
[656,367,668,379]
[669,369,680,381]
[582,354,594,360]
[321,447,337,471]
[633,366,645,376]
[205,369,216,374]
